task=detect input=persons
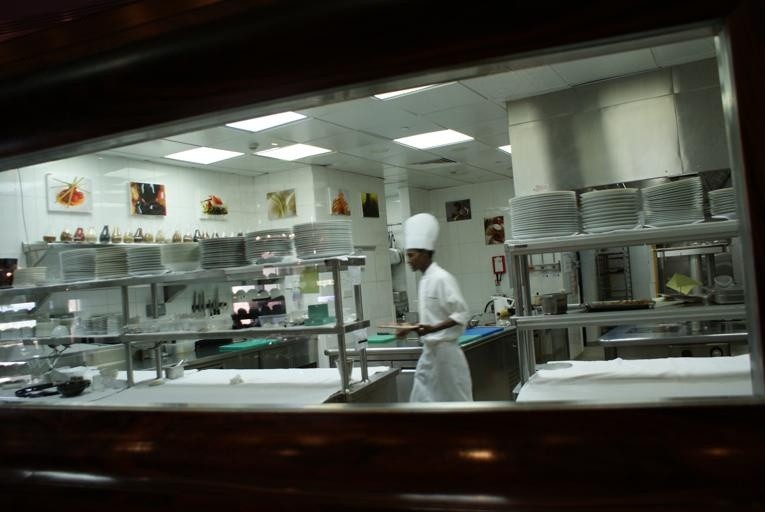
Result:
[395,249,473,401]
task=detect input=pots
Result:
[541,290,573,315]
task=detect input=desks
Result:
[325,324,515,400]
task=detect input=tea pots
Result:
[492,295,515,327]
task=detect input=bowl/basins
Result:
[234,288,283,315]
[56,380,92,397]
[102,368,118,380]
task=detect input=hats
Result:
[404,213,439,253]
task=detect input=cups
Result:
[336,358,355,382]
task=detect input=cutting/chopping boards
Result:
[367,333,400,342]
[217,337,281,352]
[455,325,507,346]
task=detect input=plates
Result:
[58,220,352,285]
[0,312,234,340]
[507,175,738,244]
[13,266,63,284]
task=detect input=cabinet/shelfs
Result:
[0,258,368,395]
[503,218,745,398]
